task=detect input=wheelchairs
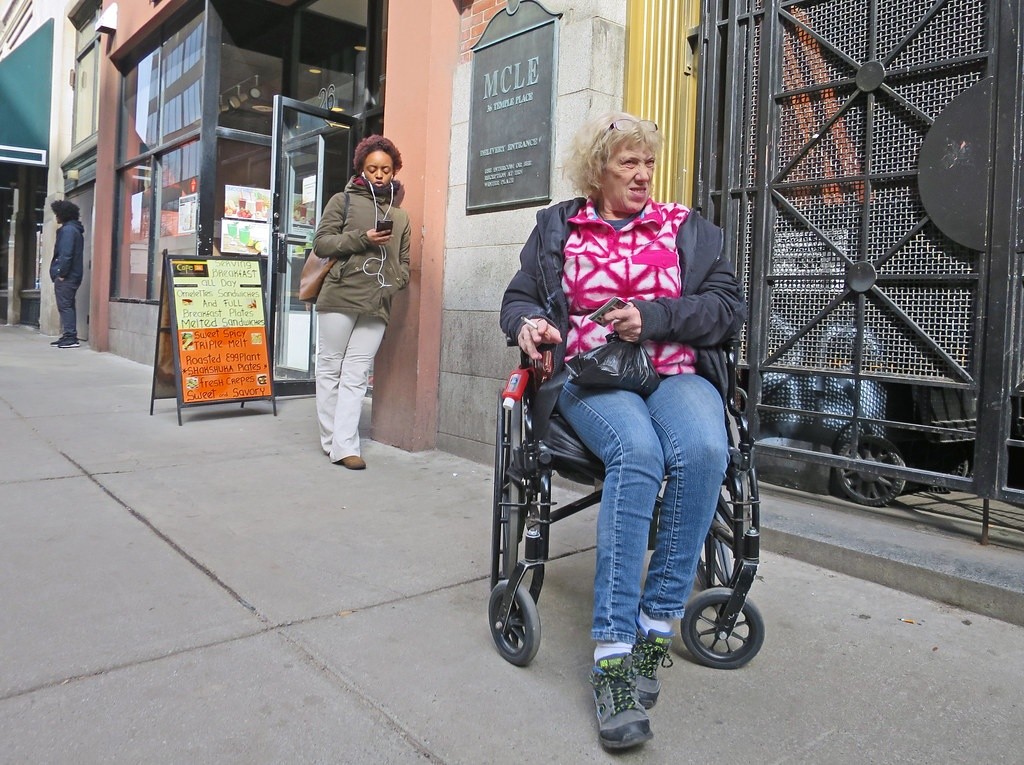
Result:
[487,335,769,670]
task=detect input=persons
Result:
[499,114,747,752]
[48,200,86,348]
[311,134,412,471]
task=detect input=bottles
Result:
[502,369,529,410]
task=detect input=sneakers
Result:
[631,617,676,709]
[590,653,654,748]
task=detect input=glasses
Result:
[601,118,659,140]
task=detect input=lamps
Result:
[249,75,267,99]
[229,85,249,109]
[67,170,78,181]
[219,95,229,114]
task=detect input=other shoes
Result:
[51,337,67,347]
[58,337,80,348]
[342,455,367,469]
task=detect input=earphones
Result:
[361,172,366,180]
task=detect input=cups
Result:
[238,197,246,210]
[256,201,263,212]
[228,224,237,237]
[238,228,250,246]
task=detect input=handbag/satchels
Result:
[299,192,349,304]
[566,327,659,394]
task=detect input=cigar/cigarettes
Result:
[520,317,551,341]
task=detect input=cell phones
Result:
[375,220,393,236]
